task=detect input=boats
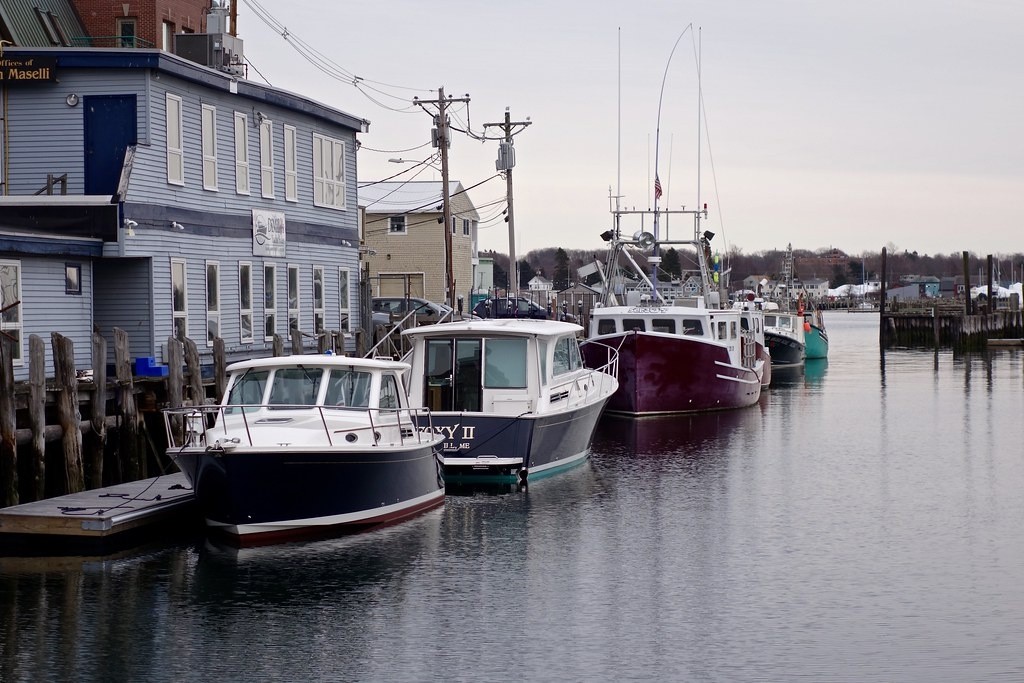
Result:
[1008,262,1024,310]
[758,242,829,358]
[162,350,446,549]
[398,317,620,492]
[970,257,1010,301]
[582,25,773,420]
[730,289,807,364]
[856,301,875,310]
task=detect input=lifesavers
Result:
[798,292,805,313]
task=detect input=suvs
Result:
[487,297,566,321]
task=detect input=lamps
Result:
[172,221,184,230]
[600,231,613,241]
[124,219,138,226]
[701,230,715,244]
[341,239,351,247]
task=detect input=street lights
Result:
[388,159,455,309]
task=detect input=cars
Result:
[370,296,483,335]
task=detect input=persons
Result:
[513,306,519,318]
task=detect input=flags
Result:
[655,175,662,199]
